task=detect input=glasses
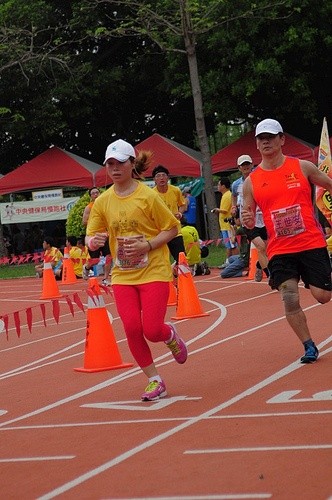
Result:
[92,192,99,195]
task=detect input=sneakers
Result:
[301,342,319,362]
[141,380,167,401]
[164,325,187,364]
[254,260,262,282]
[101,279,109,286]
[82,264,90,280]
[218,262,230,269]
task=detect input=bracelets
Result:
[88,237,99,250]
[232,206,236,209]
[147,241,152,251]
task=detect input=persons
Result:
[196,238,210,275]
[82,187,112,287]
[86,139,188,401]
[241,119,332,362]
[173,219,201,276]
[212,177,241,268]
[152,165,187,277]
[230,155,276,290]
[181,186,197,226]
[34,237,64,278]
[221,210,251,278]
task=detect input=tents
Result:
[0,144,103,228]
[77,240,94,275]
[202,129,332,175]
[94,133,208,240]
[55,236,83,279]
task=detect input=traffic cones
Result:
[169,251,210,321]
[39,254,67,299]
[74,277,134,373]
[167,282,178,306]
[60,246,80,284]
[243,242,267,279]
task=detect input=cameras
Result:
[223,215,235,226]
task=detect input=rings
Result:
[129,245,130,248]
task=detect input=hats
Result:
[103,139,136,165]
[237,155,252,166]
[182,187,191,194]
[255,119,283,137]
